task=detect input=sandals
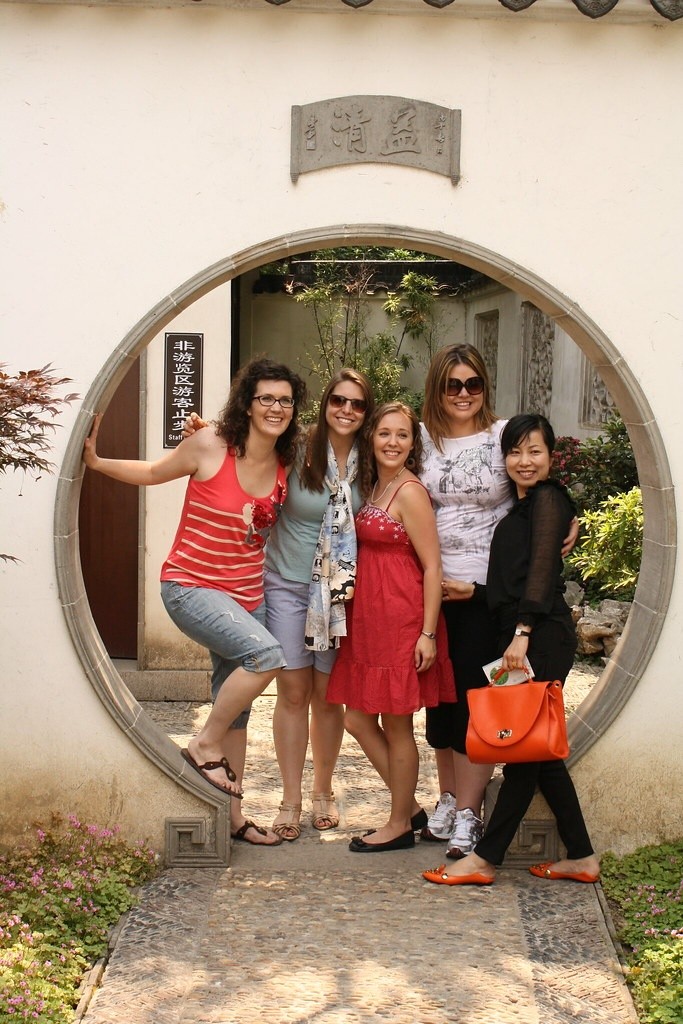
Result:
[273,800,302,840]
[310,791,339,830]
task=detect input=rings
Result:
[512,662,516,666]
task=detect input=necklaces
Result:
[371,467,406,504]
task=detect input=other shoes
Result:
[424,864,495,885]
[529,863,598,883]
[363,807,428,837]
[350,829,415,851]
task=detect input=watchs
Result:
[515,628,530,636]
[422,631,437,639]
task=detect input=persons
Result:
[325,403,457,852]
[83,359,310,845]
[423,414,602,884]
[419,343,579,860]
[181,369,375,840]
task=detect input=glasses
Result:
[251,394,295,407]
[440,376,485,397]
[329,394,367,412]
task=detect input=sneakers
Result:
[420,791,457,840]
[446,807,482,858]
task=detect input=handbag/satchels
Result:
[465,661,569,764]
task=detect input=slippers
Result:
[181,747,245,799]
[230,820,282,845]
